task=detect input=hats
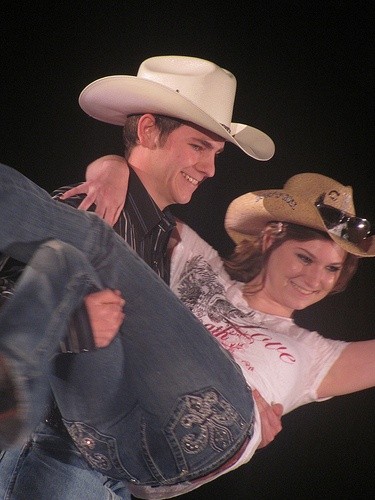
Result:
[224,172,375,258]
[78,55,276,160]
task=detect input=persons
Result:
[0,55,283,500]
[0,163,375,500]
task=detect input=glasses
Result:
[315,204,372,252]
